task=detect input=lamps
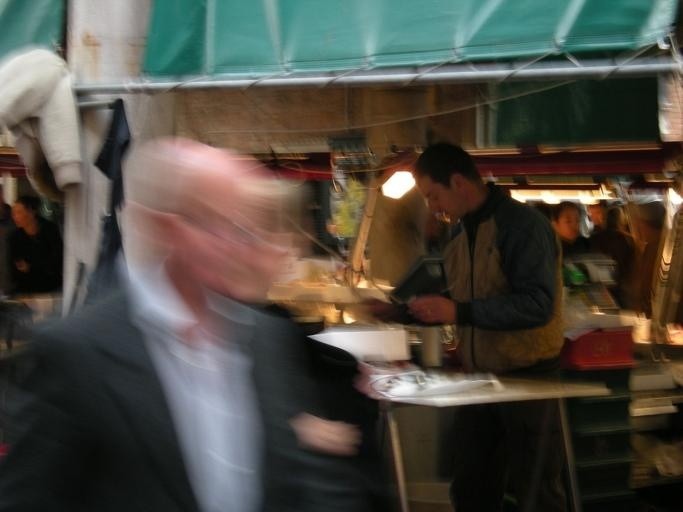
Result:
[495,184,608,206]
[377,161,418,199]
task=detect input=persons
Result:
[402,141,682,511]
[1,136,401,511]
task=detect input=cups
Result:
[418,324,443,369]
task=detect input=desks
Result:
[355,358,613,511]
